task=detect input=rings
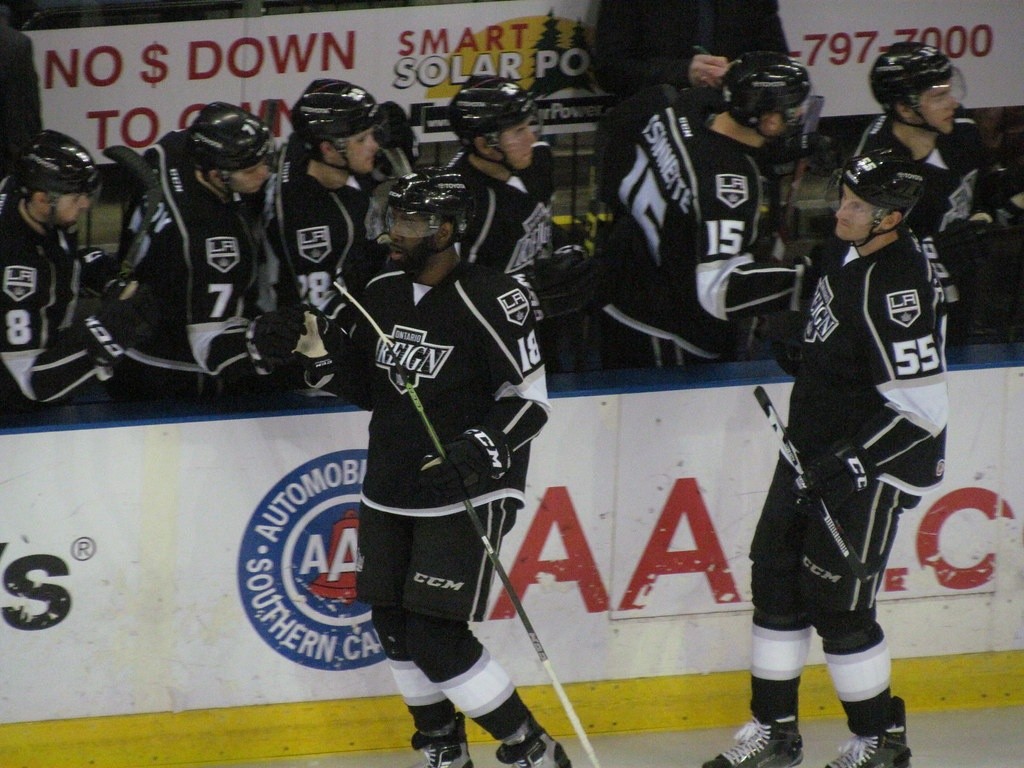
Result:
[700,76,707,81]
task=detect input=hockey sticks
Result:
[331,282,600,768]
[752,386,880,581]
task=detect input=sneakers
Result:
[823,696,912,768]
[495,714,572,768]
[702,712,804,768]
[411,713,475,768]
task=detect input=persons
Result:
[117,101,307,375]
[854,41,1024,345]
[589,51,833,363]
[243,77,415,335]
[592,0,790,185]
[445,75,592,361]
[277,165,573,768]
[0,128,152,403]
[702,148,946,768]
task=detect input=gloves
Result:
[315,293,375,378]
[776,435,868,520]
[47,245,120,292]
[420,425,513,507]
[247,310,305,358]
[71,301,151,366]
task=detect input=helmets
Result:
[289,77,380,140]
[837,147,924,232]
[180,100,270,170]
[870,42,953,115]
[11,129,101,194]
[447,75,534,141]
[721,51,812,122]
[386,164,480,242]
